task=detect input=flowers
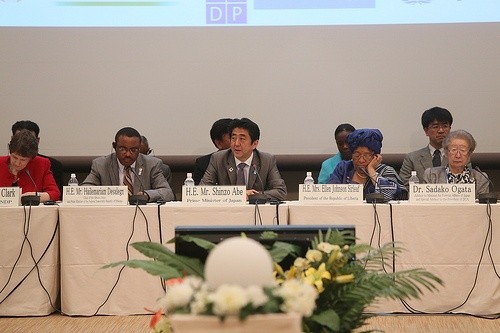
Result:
[100,228,445,333]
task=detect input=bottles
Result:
[68,173,78,186]
[408,170,419,185]
[185,173,194,186]
[304,172,314,185]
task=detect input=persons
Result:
[0,132,60,202]
[192,118,235,185]
[399,107,454,187]
[329,128,408,200]
[198,118,288,202]
[317,123,356,183]
[140,135,172,189]
[419,130,489,199]
[79,126,174,203]
[12,120,63,201]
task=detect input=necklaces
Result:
[356,171,368,178]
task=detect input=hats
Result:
[346,129,383,154]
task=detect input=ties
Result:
[123,167,134,197]
[432,150,441,167]
[236,163,246,185]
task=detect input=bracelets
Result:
[371,172,378,177]
[36,191,40,196]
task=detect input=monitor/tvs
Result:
[175,225,356,279]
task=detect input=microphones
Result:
[475,166,498,203]
[358,166,385,203]
[248,165,267,204]
[128,167,148,205]
[21,170,40,206]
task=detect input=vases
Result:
[171,312,301,333]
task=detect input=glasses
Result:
[446,148,469,154]
[427,124,451,131]
[115,144,139,153]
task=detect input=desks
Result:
[60,201,288,316]
[289,200,500,315]
[0,203,60,316]
